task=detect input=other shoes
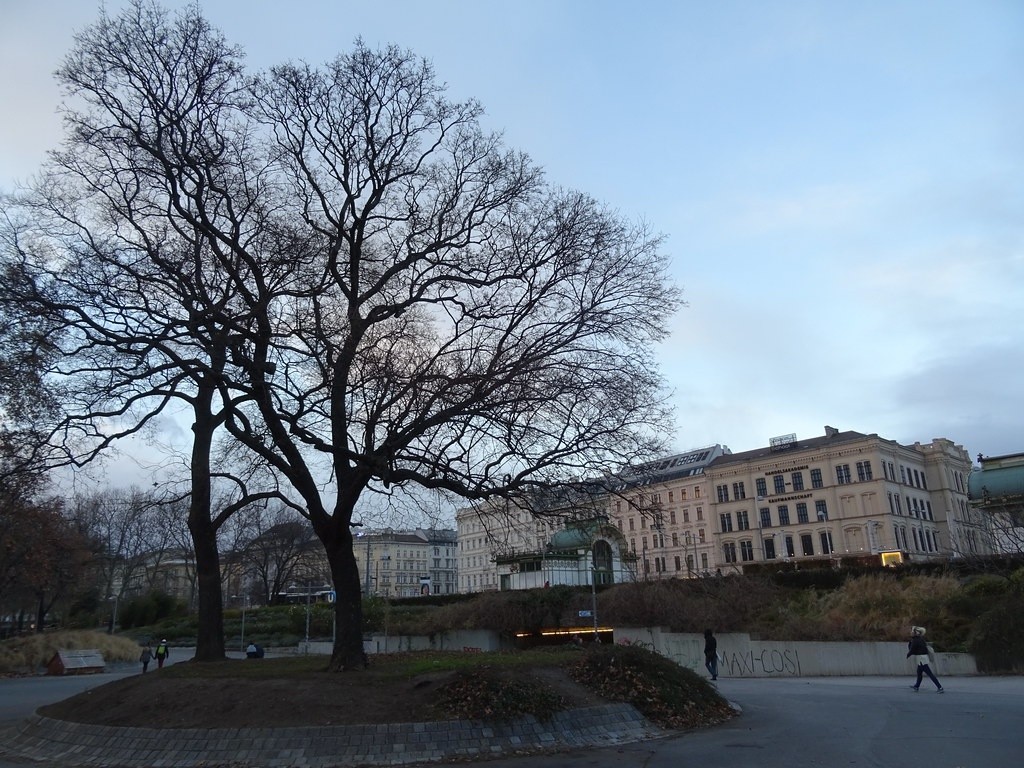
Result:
[712,674,717,679]
[936,688,944,693]
[710,678,716,680]
[910,685,919,691]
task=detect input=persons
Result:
[906,626,945,695]
[703,628,719,681]
[140,642,154,673]
[544,581,549,589]
[245,639,265,658]
[715,568,722,577]
[154,639,169,669]
[421,583,429,595]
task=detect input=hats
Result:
[162,639,166,642]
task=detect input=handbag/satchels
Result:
[927,645,935,662]
